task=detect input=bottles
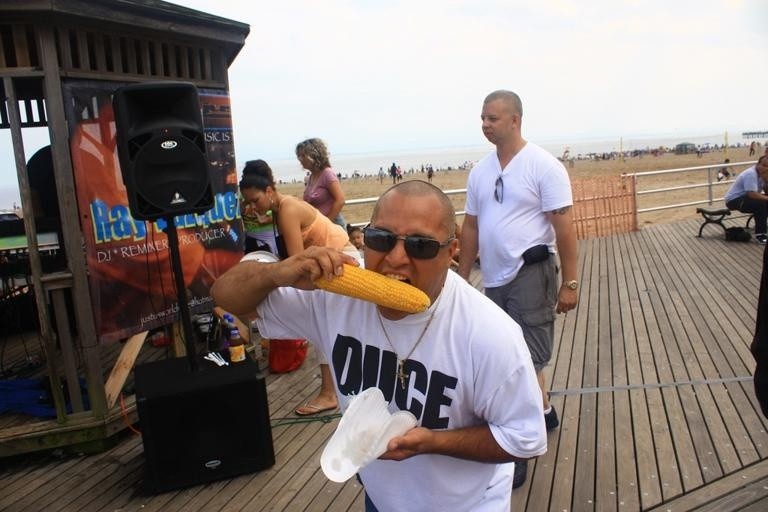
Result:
[206,309,248,367]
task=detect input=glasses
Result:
[362,221,455,260]
[493,176,504,204]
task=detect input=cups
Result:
[257,214,267,227]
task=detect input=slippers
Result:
[295,401,340,416]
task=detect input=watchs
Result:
[563,280,578,290]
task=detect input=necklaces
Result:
[377,296,441,390]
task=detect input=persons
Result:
[346,223,362,249]
[717,158,734,181]
[211,178,549,512]
[749,141,755,156]
[333,163,435,182]
[239,159,361,416]
[696,145,701,157]
[295,138,345,229]
[725,155,768,244]
[456,90,579,428]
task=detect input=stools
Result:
[696,207,754,237]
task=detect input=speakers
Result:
[111,81,217,222]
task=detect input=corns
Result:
[315,263,431,314]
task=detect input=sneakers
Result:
[544,405,559,429]
[513,459,527,489]
[755,233,767,245]
